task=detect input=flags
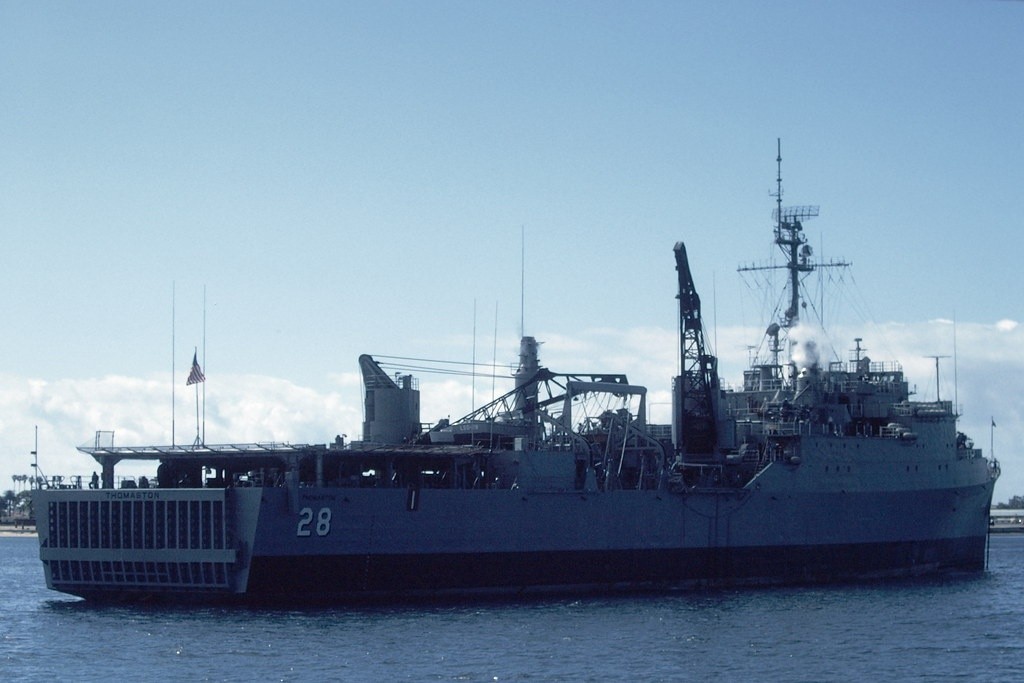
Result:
[186,347,206,386]
[992,417,996,427]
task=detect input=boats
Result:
[29,136,1000,607]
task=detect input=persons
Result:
[958,442,966,460]
[226,476,274,489]
[139,477,149,489]
[179,474,192,487]
[89,471,98,489]
